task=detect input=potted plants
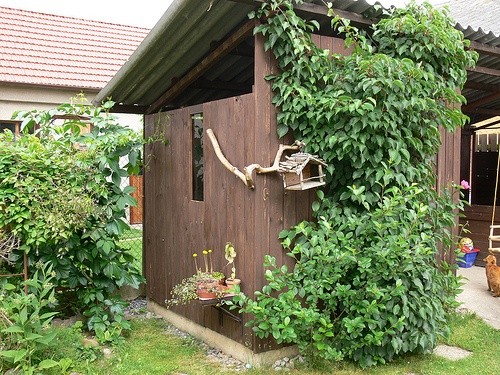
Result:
[164,248,242,308]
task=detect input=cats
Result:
[483,254,500,296]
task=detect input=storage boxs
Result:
[456,247,480,268]
